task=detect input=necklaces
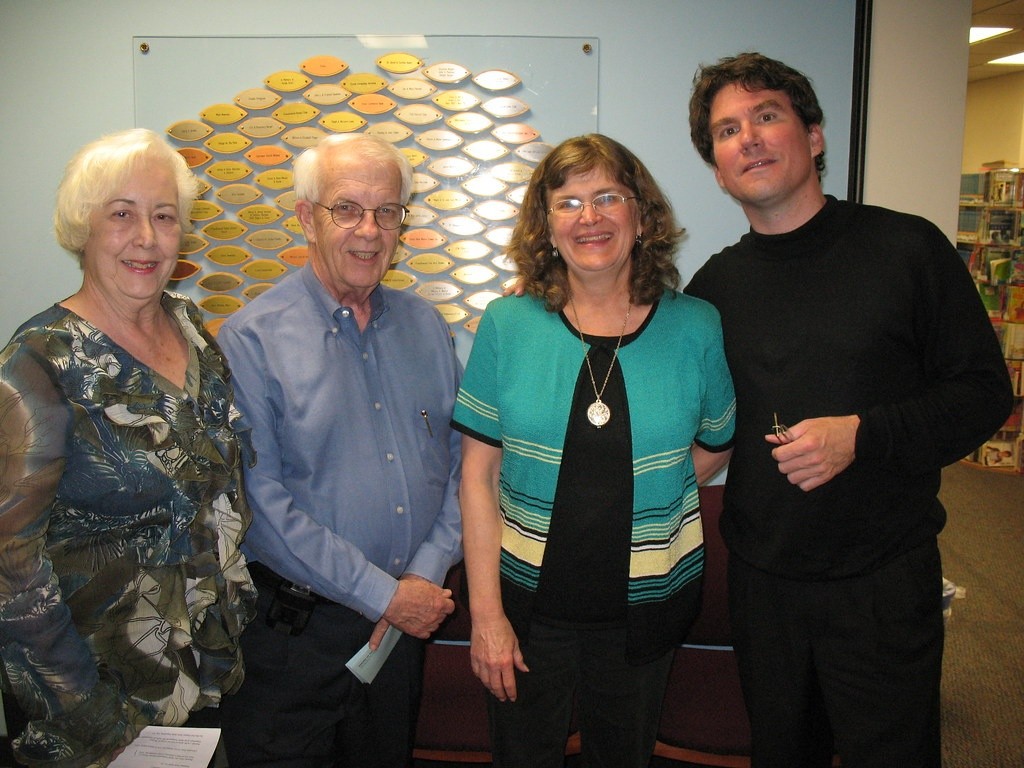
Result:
[566,291,632,427]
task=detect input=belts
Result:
[246,561,339,608]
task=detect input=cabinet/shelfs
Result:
[952,169,1024,474]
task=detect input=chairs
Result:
[410,481,842,768]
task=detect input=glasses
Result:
[546,193,635,218]
[315,201,409,230]
[771,413,795,445]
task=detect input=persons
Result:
[0,129,257,768]
[504,54,1013,768]
[448,136,736,768]
[214,133,465,768]
